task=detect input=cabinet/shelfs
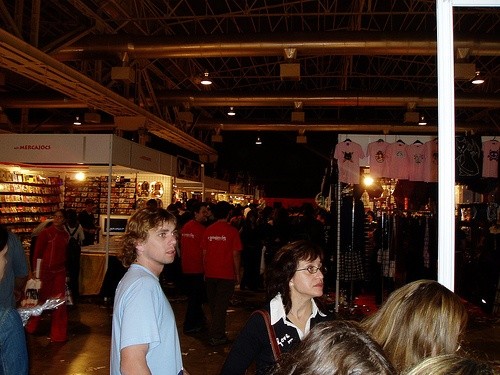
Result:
[0,172,138,256]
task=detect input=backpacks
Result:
[65,225,80,253]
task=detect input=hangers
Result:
[434,136,438,140]
[397,135,406,144]
[343,134,352,142]
[490,136,497,144]
[413,137,423,147]
[376,139,384,143]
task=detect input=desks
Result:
[76,235,129,306]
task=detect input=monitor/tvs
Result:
[102,217,128,235]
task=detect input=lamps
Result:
[201,69,213,85]
[110,51,136,85]
[84,107,101,124]
[175,102,194,123]
[211,127,224,143]
[296,128,307,144]
[455,47,476,82]
[472,68,485,85]
[417,113,428,126]
[279,48,301,82]
[403,101,420,124]
[291,101,305,122]
[227,106,236,117]
[255,136,262,144]
[73,114,82,126]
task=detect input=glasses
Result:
[296,266,328,274]
[146,229,179,240]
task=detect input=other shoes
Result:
[183,327,206,334]
[209,336,229,345]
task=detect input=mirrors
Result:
[149,180,164,199]
[136,181,150,197]
[155,199,163,209]
[136,197,150,209]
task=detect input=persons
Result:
[0,226,9,280]
[147,199,324,347]
[270,279,494,375]
[24,198,100,342]
[218,240,331,375]
[0,232,33,375]
[109,205,190,375]
